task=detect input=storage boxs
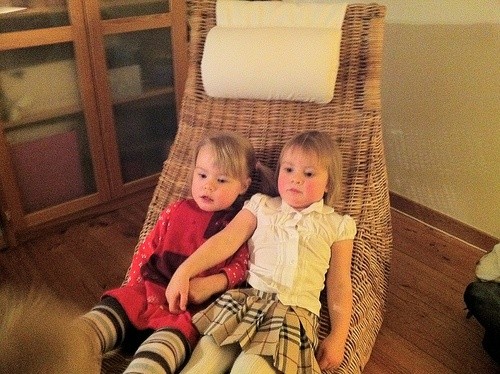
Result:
[106,65,144,100]
[0,60,81,118]
[5,121,85,212]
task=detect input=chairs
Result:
[120,0,392,374]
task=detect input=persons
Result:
[165,130,356,374]
[80,131,278,374]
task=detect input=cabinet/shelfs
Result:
[0,0,191,245]
